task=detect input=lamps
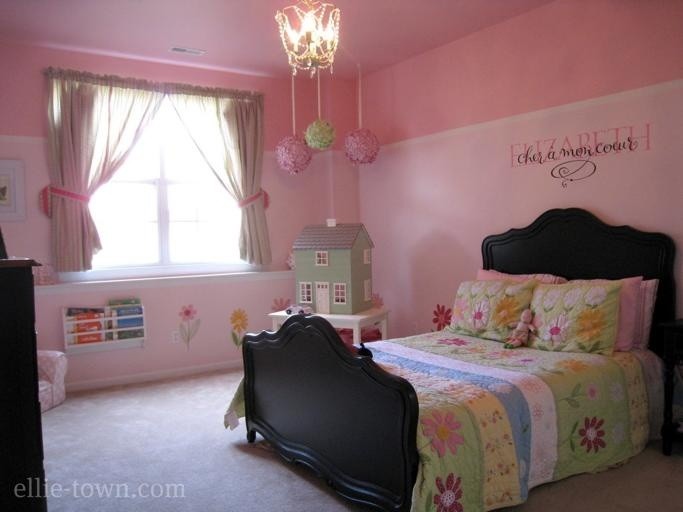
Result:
[274,1,341,79]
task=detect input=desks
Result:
[266,308,388,346]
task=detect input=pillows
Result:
[449,269,659,354]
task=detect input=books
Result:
[63,297,144,346]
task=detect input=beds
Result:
[242,207,677,510]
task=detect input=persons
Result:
[503,307,536,349]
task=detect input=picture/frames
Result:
[0,159,26,222]
